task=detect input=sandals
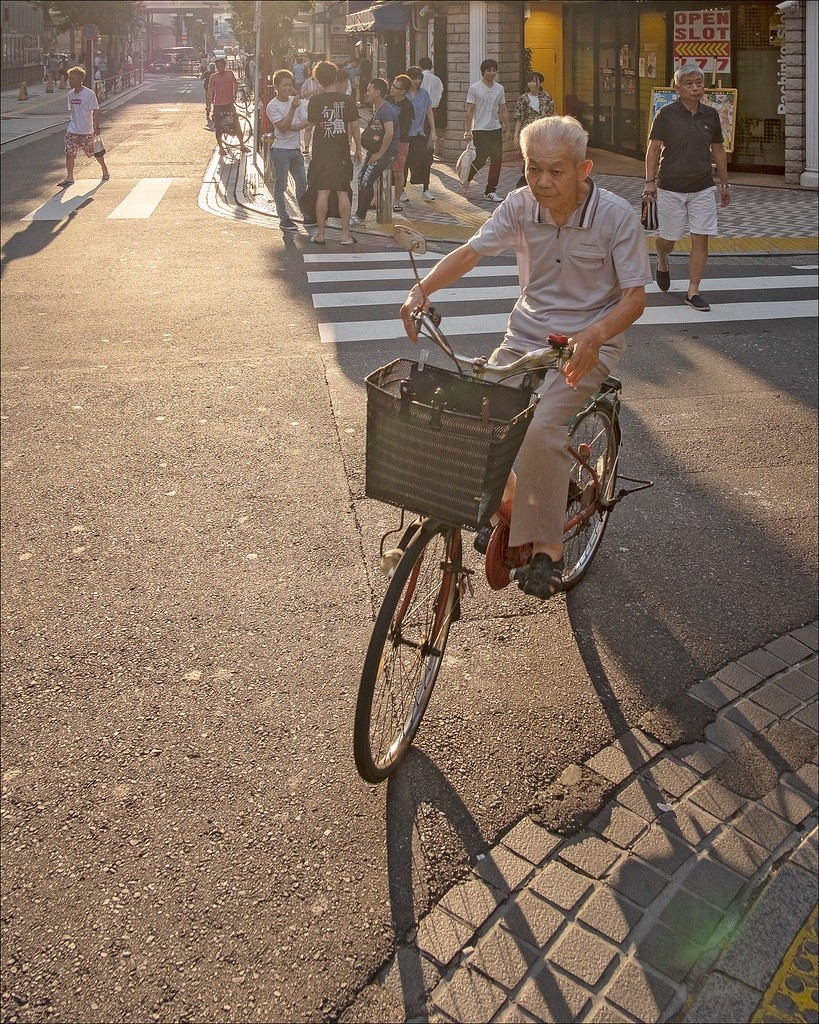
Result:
[473,529,492,554]
[517,552,565,600]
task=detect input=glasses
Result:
[392,83,401,90]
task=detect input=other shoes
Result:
[350,216,364,225]
[655,257,670,291]
[684,294,710,311]
[302,151,309,154]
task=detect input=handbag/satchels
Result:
[301,99,309,112]
[361,127,385,153]
[641,192,659,231]
[93,135,106,157]
[456,143,476,183]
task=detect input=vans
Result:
[162,47,196,63]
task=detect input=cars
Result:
[223,45,239,56]
[148,54,177,74]
[212,50,228,61]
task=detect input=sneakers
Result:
[400,192,409,201]
[422,190,435,201]
[280,219,298,229]
[303,219,318,226]
[483,192,504,202]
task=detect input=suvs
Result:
[43,53,68,69]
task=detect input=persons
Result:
[641,64,732,312]
[401,115,652,599]
[264,69,328,230]
[350,78,398,224]
[460,59,514,204]
[513,72,558,189]
[41,45,444,212]
[301,61,363,246]
[207,58,251,155]
[55,67,110,186]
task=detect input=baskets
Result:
[362,358,541,530]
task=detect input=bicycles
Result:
[351,224,655,786]
[234,76,251,112]
[221,113,253,147]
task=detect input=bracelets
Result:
[645,179,655,183]
[719,184,730,189]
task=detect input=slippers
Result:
[340,236,358,244]
[393,203,402,211]
[219,150,227,155]
[57,180,73,185]
[102,175,109,180]
[311,236,325,244]
[240,147,251,152]
